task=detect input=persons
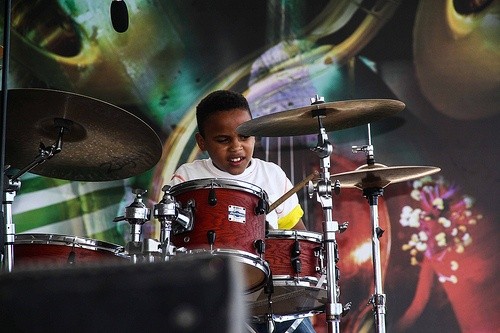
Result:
[168,90,317,333]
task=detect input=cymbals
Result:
[304,163,442,189]
[234,98,406,138]
[0,87,163,182]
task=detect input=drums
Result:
[247,228,328,324]
[0,233,131,273]
[159,177,271,296]
